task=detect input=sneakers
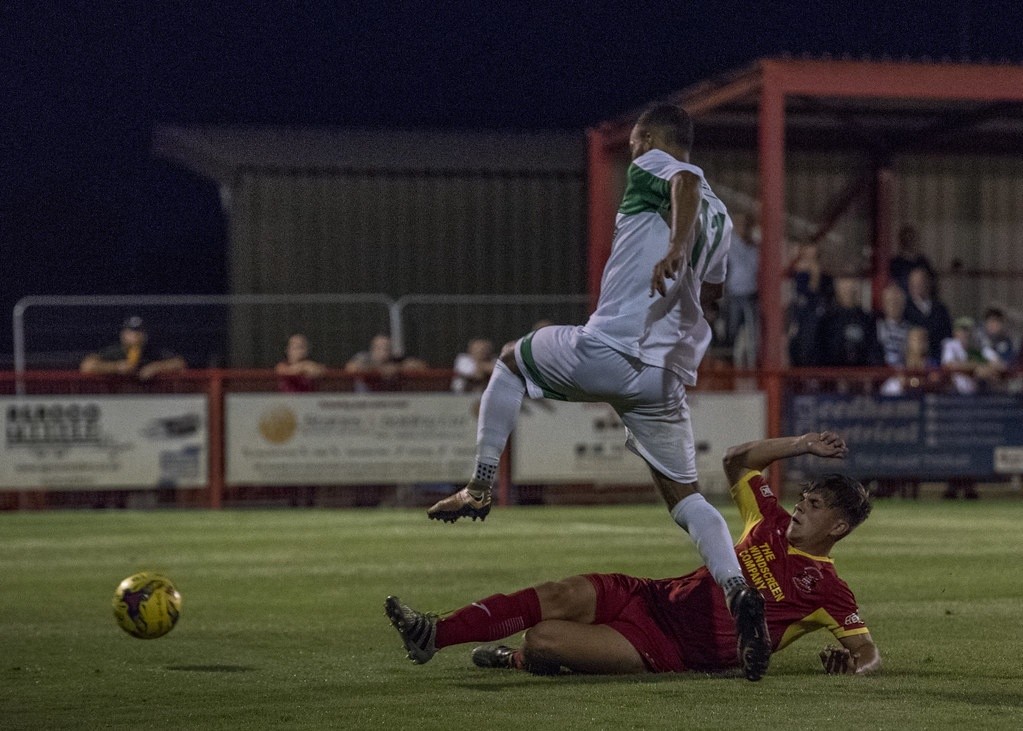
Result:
[725,586,772,681]
[383,596,454,667]
[426,482,491,523]
[470,643,521,669]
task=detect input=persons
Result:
[710,223,1023,499]
[346,334,422,508]
[450,334,496,494]
[390,431,879,674]
[77,315,186,509]
[276,332,323,507]
[428,107,772,680]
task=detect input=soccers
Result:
[112,573,181,638]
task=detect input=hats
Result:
[123,316,148,333]
